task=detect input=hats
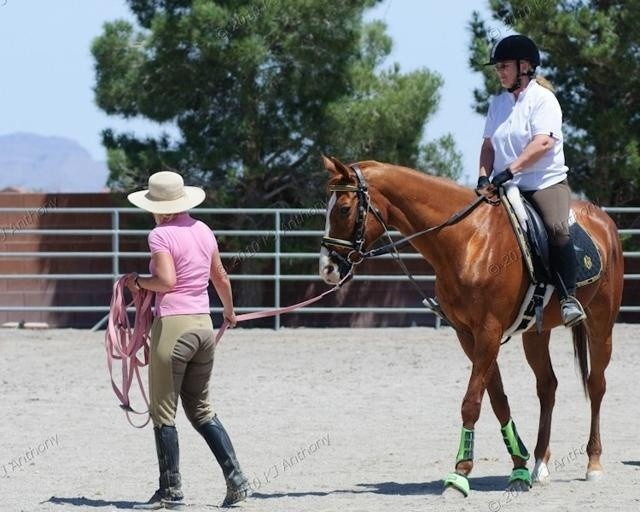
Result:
[128,172,207,214]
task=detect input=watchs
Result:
[134,276,143,291]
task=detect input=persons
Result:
[422,34,587,328]
[124,170,254,507]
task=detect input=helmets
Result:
[481,34,540,67]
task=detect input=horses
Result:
[316,150,625,504]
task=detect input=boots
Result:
[150,424,184,502]
[548,238,581,325]
[198,414,253,505]
